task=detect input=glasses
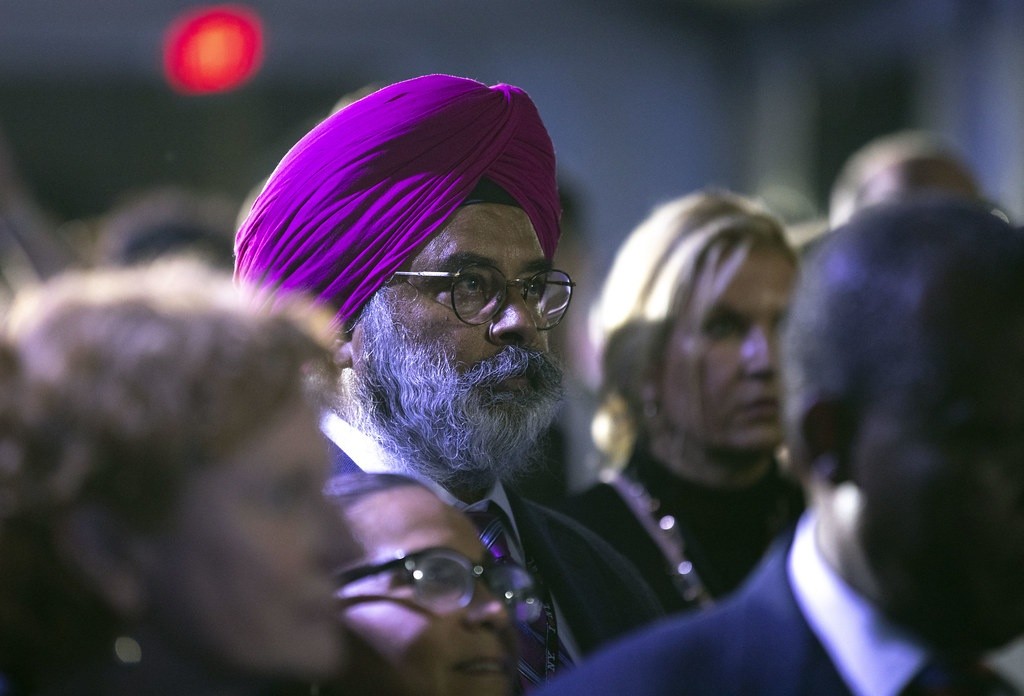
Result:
[329,548,547,625]
[391,264,576,330]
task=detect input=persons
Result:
[0,250,362,696]
[529,194,1024,696]
[86,174,598,412]
[232,72,661,696]
[322,472,519,695]
[539,191,803,620]
[828,125,987,243]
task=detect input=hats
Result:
[234,76,560,334]
